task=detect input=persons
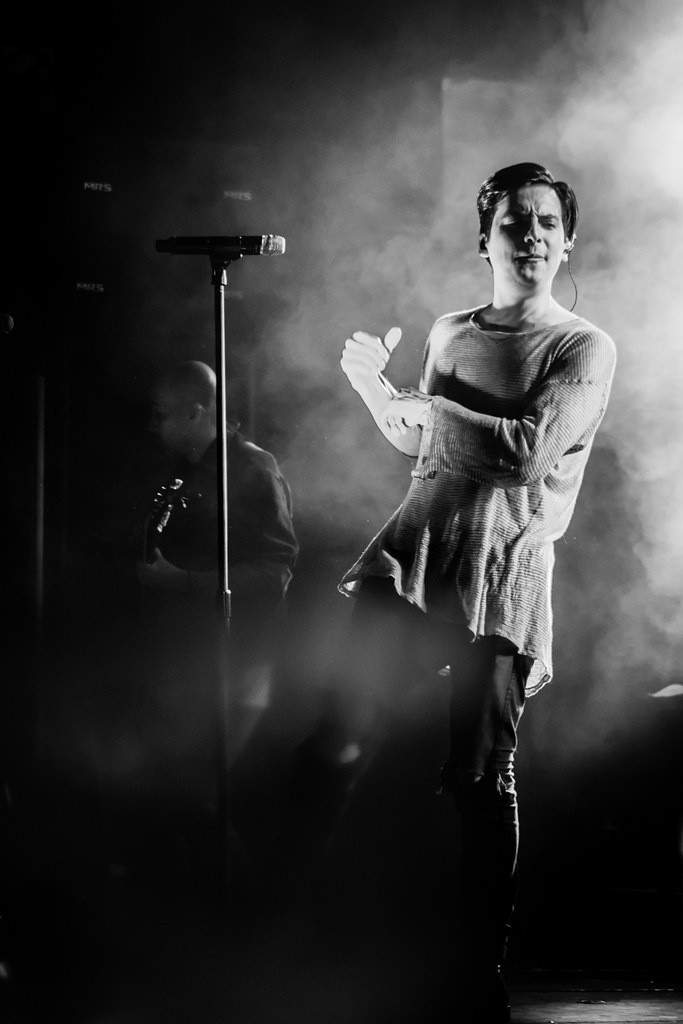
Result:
[250,162,617,1024]
[134,362,300,780]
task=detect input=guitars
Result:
[119,473,195,565]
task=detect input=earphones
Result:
[563,240,574,252]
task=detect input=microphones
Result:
[154,234,287,256]
